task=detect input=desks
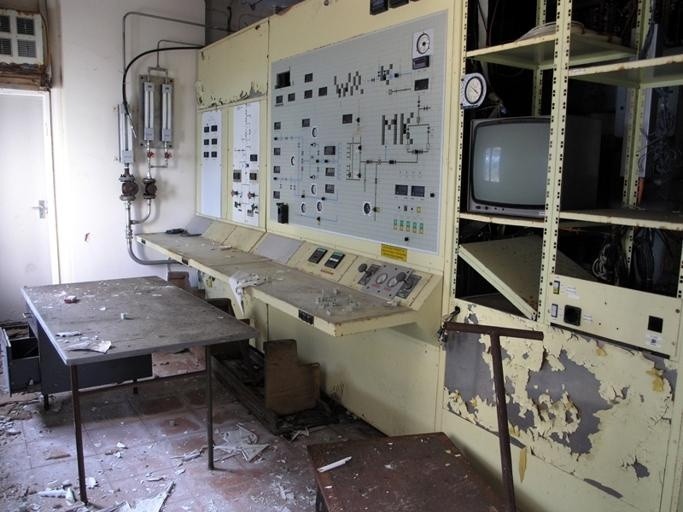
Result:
[308,432,513,512]
[1,274,262,507]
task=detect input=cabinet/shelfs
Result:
[438,1,683,509]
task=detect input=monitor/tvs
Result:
[468,116,551,219]
